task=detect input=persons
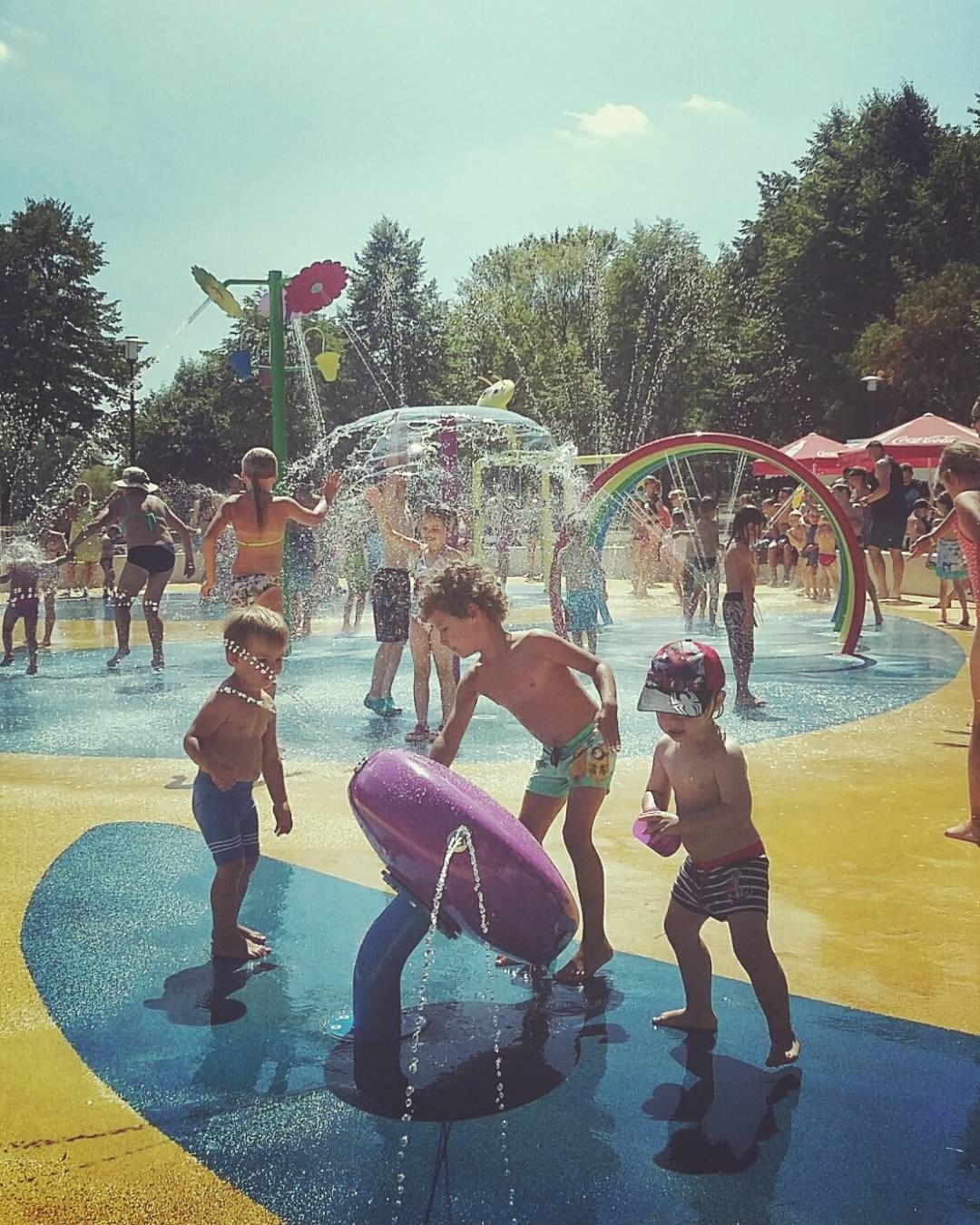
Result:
[934,441,980,844]
[182,605,293,957]
[417,563,614,981]
[630,441,970,708]
[200,446,604,741]
[0,466,197,675]
[636,640,800,1067]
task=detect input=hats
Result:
[112,466,159,493]
[636,640,725,717]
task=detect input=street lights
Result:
[112,336,147,468]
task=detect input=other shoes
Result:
[26,659,37,674]
[427,725,445,741]
[0,652,14,668]
[364,696,402,716]
[405,723,430,741]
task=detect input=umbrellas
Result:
[837,413,979,489]
[750,431,854,476]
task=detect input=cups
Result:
[633,808,681,858]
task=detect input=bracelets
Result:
[929,536,937,548]
